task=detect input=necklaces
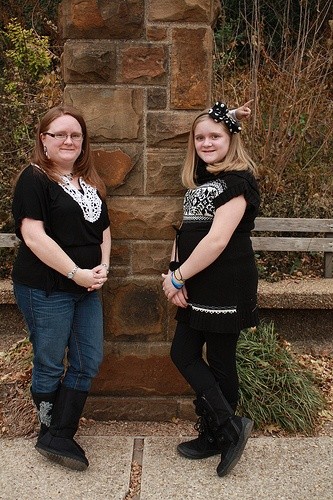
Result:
[64,173,73,180]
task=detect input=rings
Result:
[99,279,101,283]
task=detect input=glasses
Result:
[44,132,85,141]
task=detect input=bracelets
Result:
[169,262,180,271]
[171,268,185,290]
[101,264,110,270]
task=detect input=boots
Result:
[176,387,239,459]
[198,383,254,476]
[30,383,89,471]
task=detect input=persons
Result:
[10,106,112,471]
[228,99,254,121]
[161,101,259,477]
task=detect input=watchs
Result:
[68,266,78,279]
